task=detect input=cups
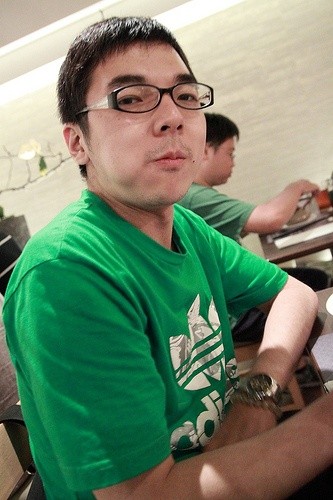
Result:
[313,181,333,213]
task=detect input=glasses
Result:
[74,81,214,116]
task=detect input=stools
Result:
[235,342,305,413]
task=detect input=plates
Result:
[325,293,333,316]
[281,213,317,229]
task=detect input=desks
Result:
[258,207,333,265]
[305,287,333,396]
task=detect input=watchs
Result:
[235,371,285,403]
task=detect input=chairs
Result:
[0,405,47,500]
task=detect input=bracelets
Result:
[229,393,284,419]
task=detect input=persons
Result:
[1,16,332,500]
[176,111,333,389]
